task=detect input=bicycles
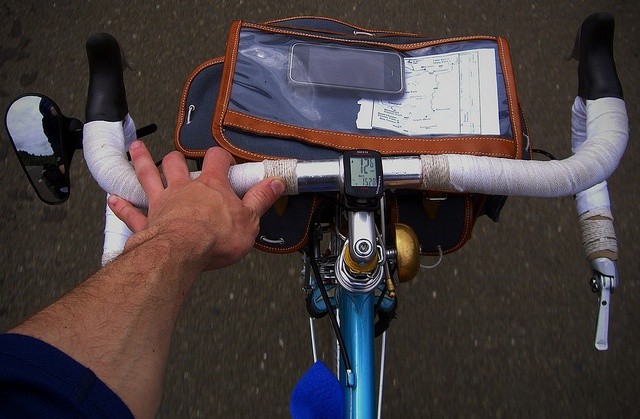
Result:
[5,11,631,418]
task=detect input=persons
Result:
[1,139,287,414]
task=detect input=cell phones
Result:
[287,42,407,102]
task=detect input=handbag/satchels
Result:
[175,15,531,255]
